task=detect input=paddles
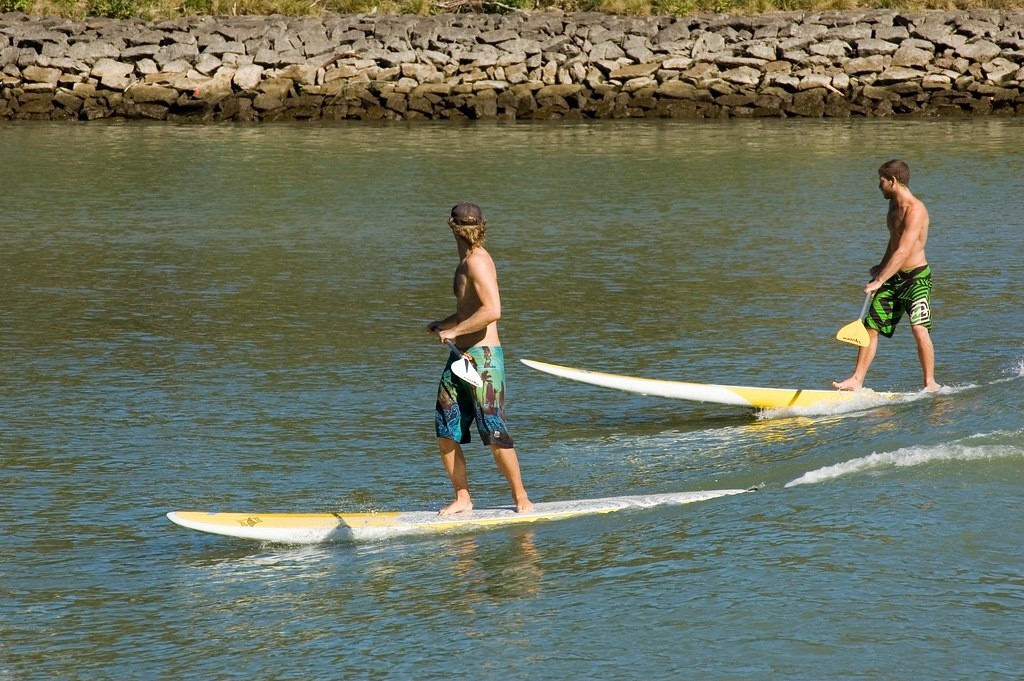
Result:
[433,325,485,390]
[836,291,871,348]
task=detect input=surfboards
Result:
[517,355,914,409]
[165,489,754,545]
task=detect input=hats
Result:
[450,202,482,225]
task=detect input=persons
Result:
[832,159,942,392]
[426,201,535,516]
